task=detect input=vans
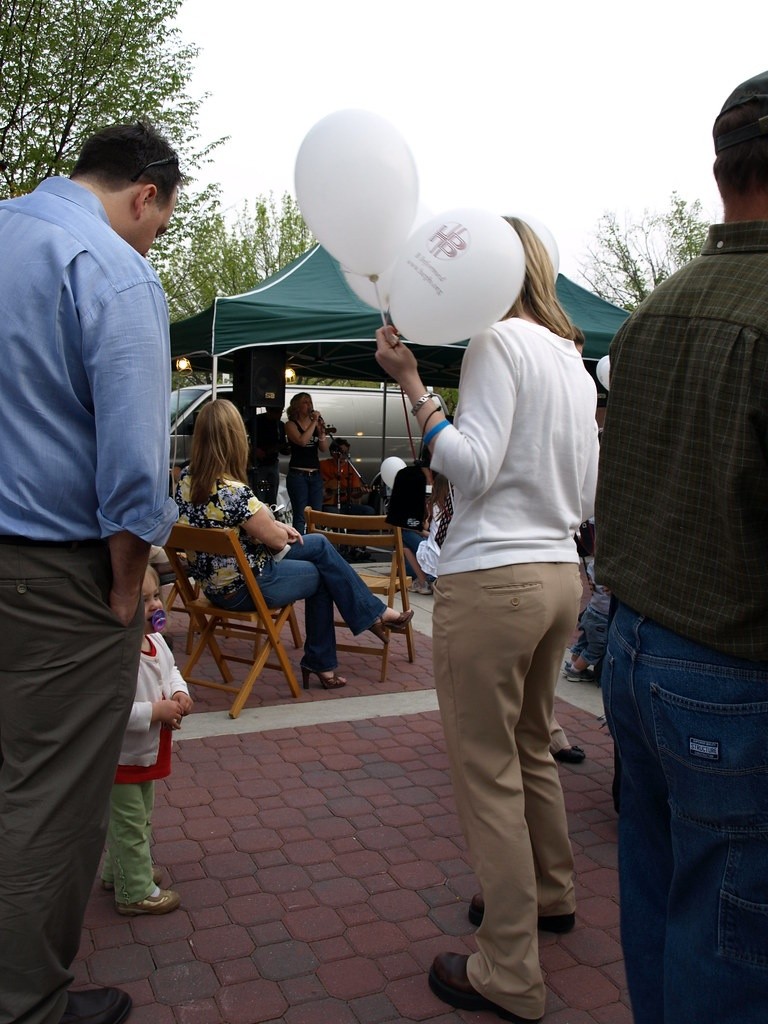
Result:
[169,383,452,512]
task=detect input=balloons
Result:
[293,108,528,347]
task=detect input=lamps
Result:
[176,359,193,377]
[285,367,296,383]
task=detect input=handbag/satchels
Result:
[385,459,426,532]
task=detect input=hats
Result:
[713,68,768,154]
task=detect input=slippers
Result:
[407,579,432,595]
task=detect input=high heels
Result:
[300,664,347,689]
[367,609,414,644]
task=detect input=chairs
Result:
[302,510,414,683]
[155,505,303,718]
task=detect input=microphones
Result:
[309,408,323,420]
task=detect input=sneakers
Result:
[561,661,595,682]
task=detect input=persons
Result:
[175,399,415,690]
[599,78,768,1024]
[319,438,376,515]
[550,710,587,764]
[561,553,618,682]
[100,561,194,916]
[372,216,601,1024]
[397,470,456,595]
[246,400,292,511]
[0,124,181,1024]
[284,392,329,535]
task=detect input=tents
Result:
[169,224,631,535]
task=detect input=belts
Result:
[0,534,99,550]
[288,468,320,477]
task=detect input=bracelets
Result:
[318,438,326,442]
[411,390,431,417]
[422,419,451,447]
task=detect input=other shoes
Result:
[114,888,180,916]
[339,550,349,559]
[351,549,372,562]
[104,867,162,891]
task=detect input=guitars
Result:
[322,479,381,505]
[279,420,337,447]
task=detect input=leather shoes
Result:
[468,892,576,934]
[428,950,545,1022]
[56,986,133,1024]
[554,745,586,764]
